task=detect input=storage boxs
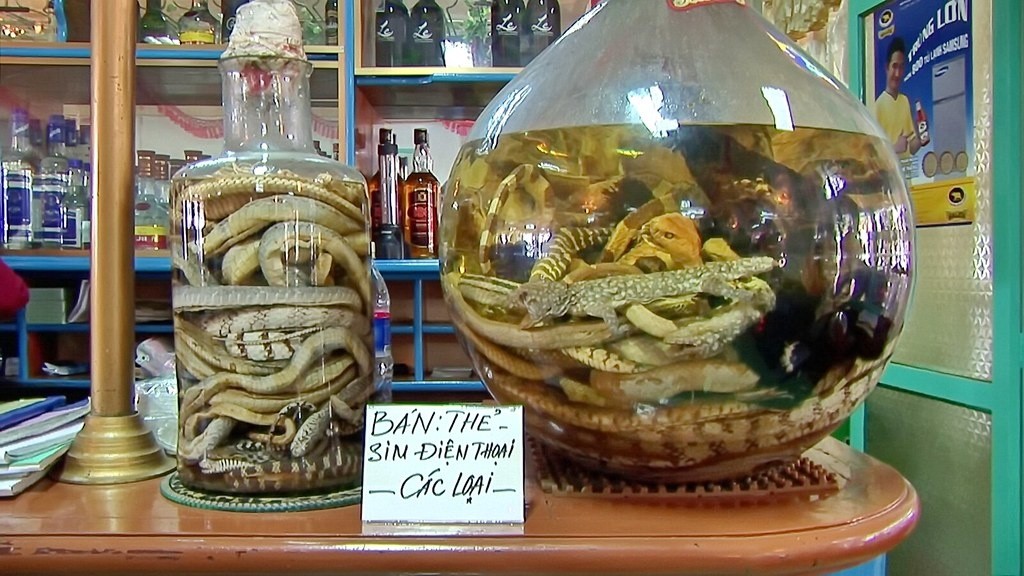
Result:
[27,288,70,324]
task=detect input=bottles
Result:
[526,0,561,66]
[60,118,91,250]
[372,266,392,404]
[33,115,69,248]
[437,0,915,482]
[170,160,185,177]
[154,154,171,249]
[0,109,34,250]
[30,119,43,150]
[178,0,220,45]
[133,150,158,249]
[325,0,338,46]
[375,0,410,67]
[159,0,372,511]
[184,150,214,163]
[410,0,446,67]
[915,100,931,146]
[491,0,526,67]
[402,128,441,259]
[140,0,168,37]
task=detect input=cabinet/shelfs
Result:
[0,0,524,393]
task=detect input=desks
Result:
[0,432,919,576]
